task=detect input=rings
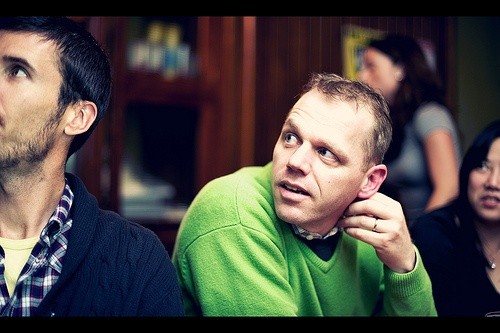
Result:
[373,219,378,231]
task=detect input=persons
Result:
[0,10,190,318]
[357,28,463,221]
[169,70,438,318]
[411,119,500,316]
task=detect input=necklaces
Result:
[477,238,500,270]
